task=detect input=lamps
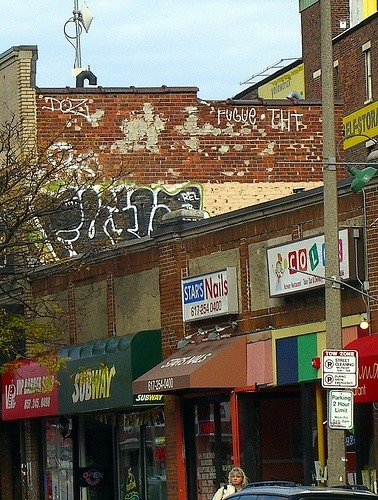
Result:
[360,318,369,330]
[337,134,378,195]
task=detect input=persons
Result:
[213,468,247,500]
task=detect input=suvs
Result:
[225,481,377,500]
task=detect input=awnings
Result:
[133,336,247,395]
[2,329,164,421]
[344,335,378,402]
